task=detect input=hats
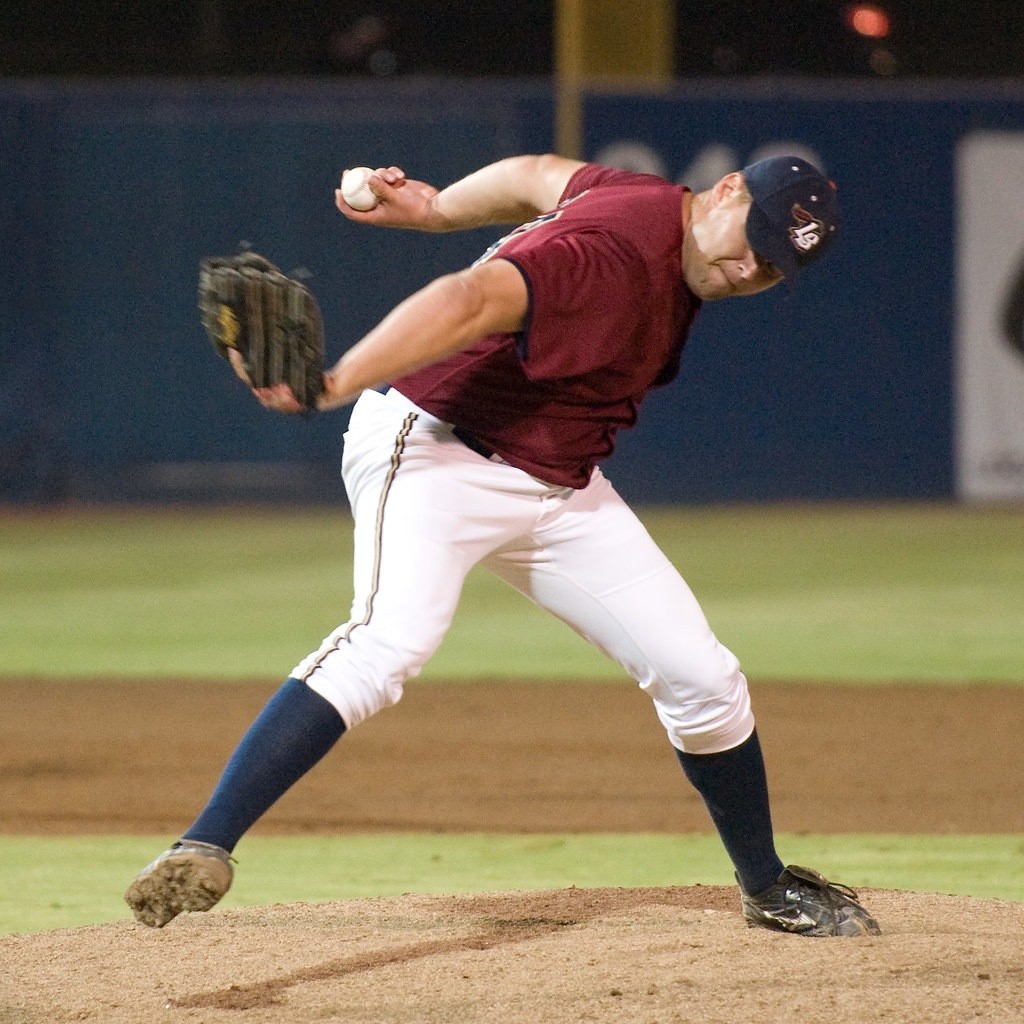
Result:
[734,156,837,283]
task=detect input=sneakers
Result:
[734,863,881,937]
[124,838,238,929]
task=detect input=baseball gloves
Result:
[196,253,331,426]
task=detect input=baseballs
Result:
[341,165,384,215]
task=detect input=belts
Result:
[370,381,493,459]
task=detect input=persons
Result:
[126,154,881,936]
